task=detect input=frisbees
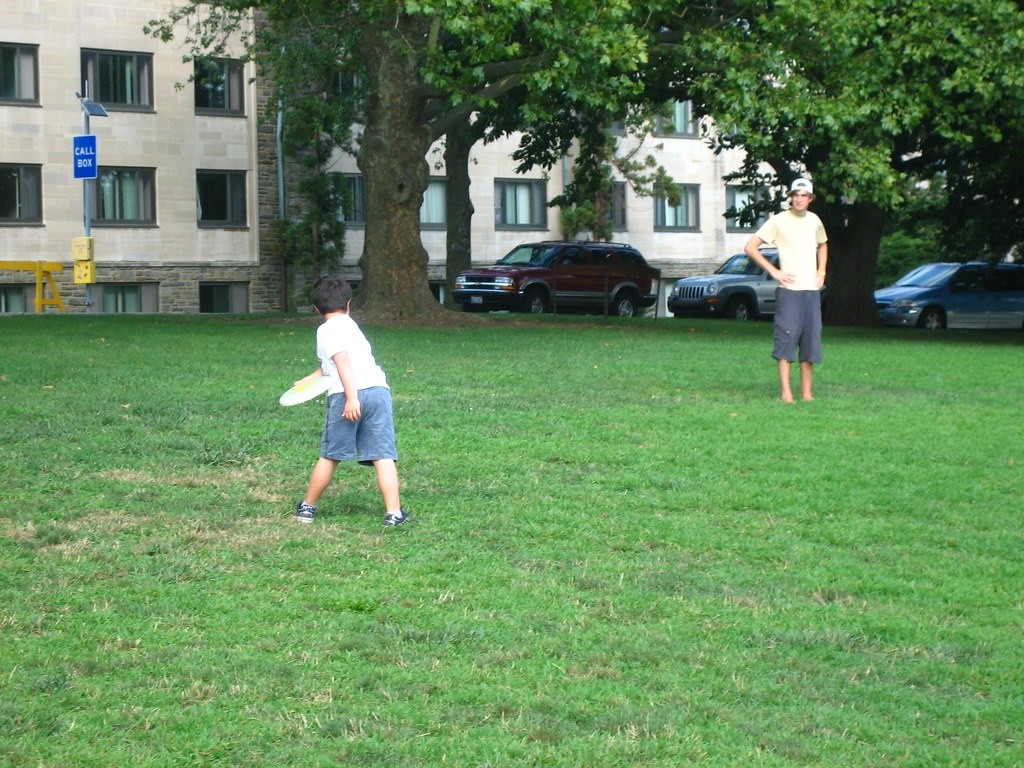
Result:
[278,376,334,407]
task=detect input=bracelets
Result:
[818,270,825,275]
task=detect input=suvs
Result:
[667,247,780,321]
[874,260,1024,331]
[450,239,657,319]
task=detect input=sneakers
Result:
[296,500,318,523]
[382,507,415,527]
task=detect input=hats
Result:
[791,178,813,194]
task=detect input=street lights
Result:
[74,80,109,313]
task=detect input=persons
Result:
[744,178,829,403]
[294,275,412,528]
